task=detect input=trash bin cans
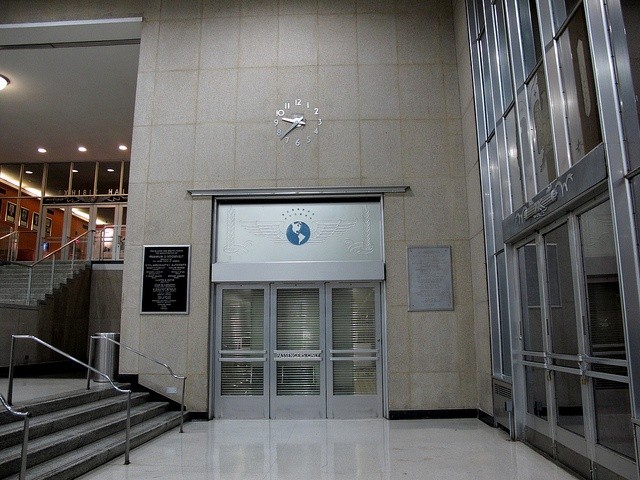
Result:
[93,332,120,383]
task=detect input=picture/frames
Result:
[406,245,454,311]
[140,244,190,315]
[46,217,52,236]
[19,206,29,228]
[31,211,39,230]
[5,201,17,224]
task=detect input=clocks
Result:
[273,99,322,146]
